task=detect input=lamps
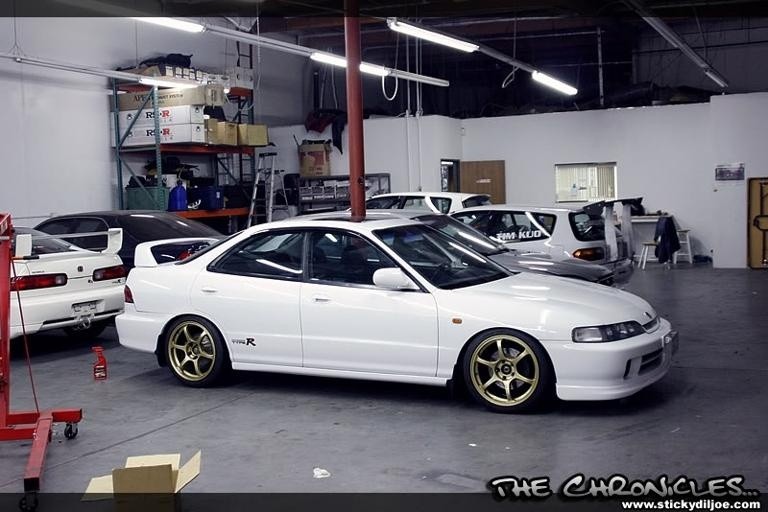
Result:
[309,51,391,76]
[531,70,578,97]
[387,19,480,54]
[128,17,205,33]
[138,78,202,88]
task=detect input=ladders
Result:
[247,152,291,228]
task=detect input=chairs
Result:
[637,216,681,271]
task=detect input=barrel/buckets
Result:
[169,181,187,212]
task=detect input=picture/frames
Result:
[714,161,746,187]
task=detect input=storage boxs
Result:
[292,133,332,178]
[79,448,200,512]
[109,66,270,148]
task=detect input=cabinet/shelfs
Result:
[112,76,257,234]
[283,173,391,215]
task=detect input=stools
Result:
[672,230,694,265]
